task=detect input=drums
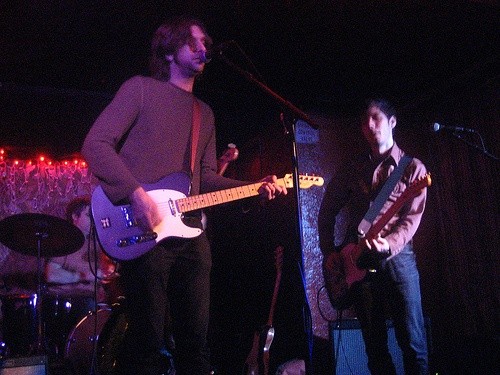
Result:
[62,307,133,375]
[0,293,97,369]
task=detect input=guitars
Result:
[242,245,286,375]
[321,171,432,312]
[90,173,325,263]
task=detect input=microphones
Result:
[430,123,477,135]
[196,40,232,65]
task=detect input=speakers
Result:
[330,316,437,375]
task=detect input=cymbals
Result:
[0,213,86,258]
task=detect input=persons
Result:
[318,97,428,375]
[44,197,106,285]
[80,18,289,375]
